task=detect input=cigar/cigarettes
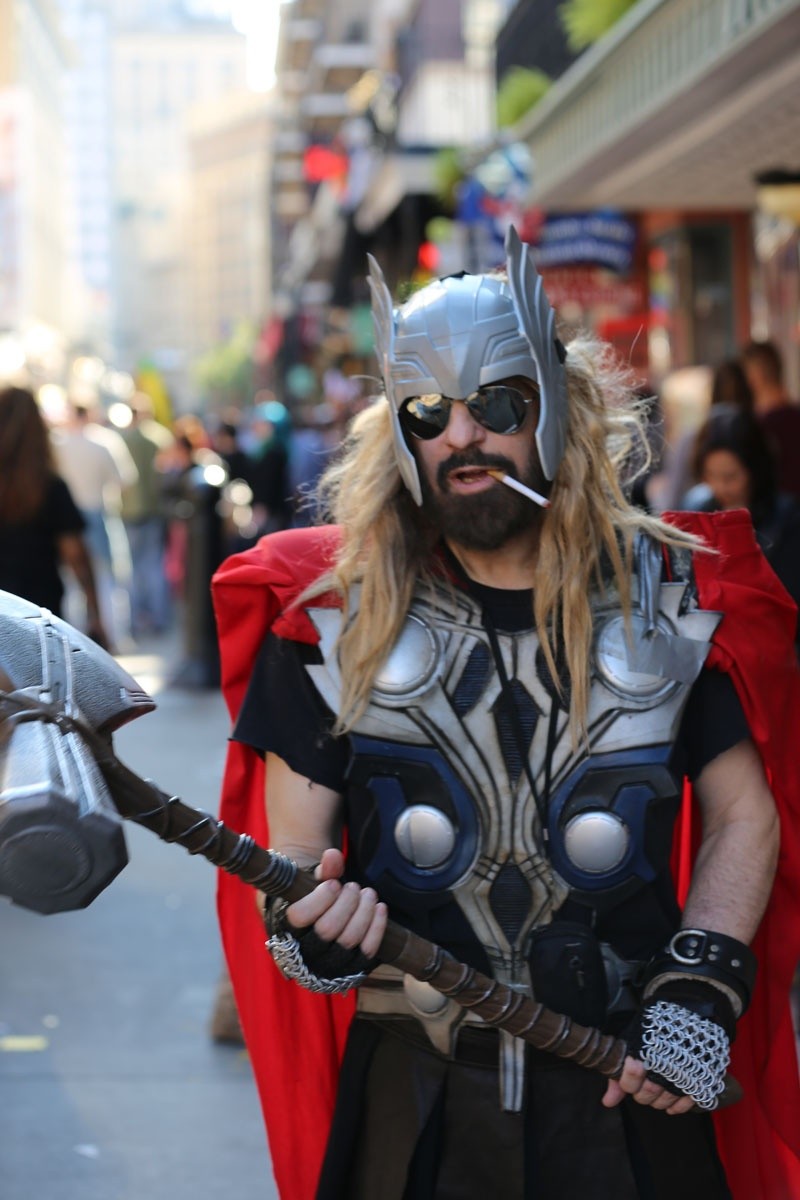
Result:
[485,468,553,508]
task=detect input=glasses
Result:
[400,384,536,441]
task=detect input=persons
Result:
[213,224,800,1200]
[0,268,799,686]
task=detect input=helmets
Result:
[366,225,567,510]
